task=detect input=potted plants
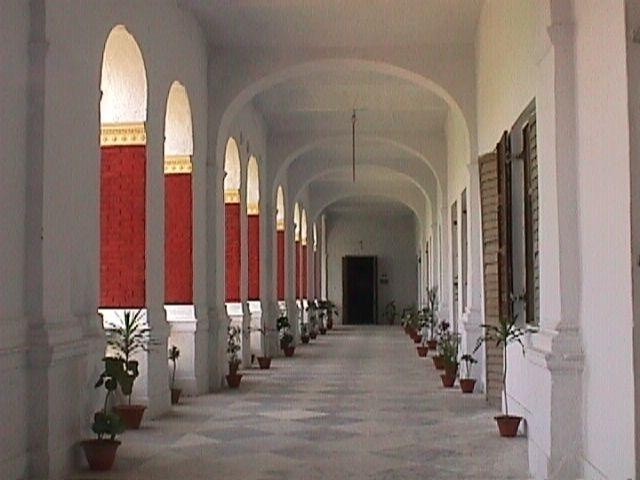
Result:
[79,358,139,472]
[381,286,477,394]
[225,296,339,388]
[472,312,539,437]
[167,344,182,406]
[103,307,161,431]
